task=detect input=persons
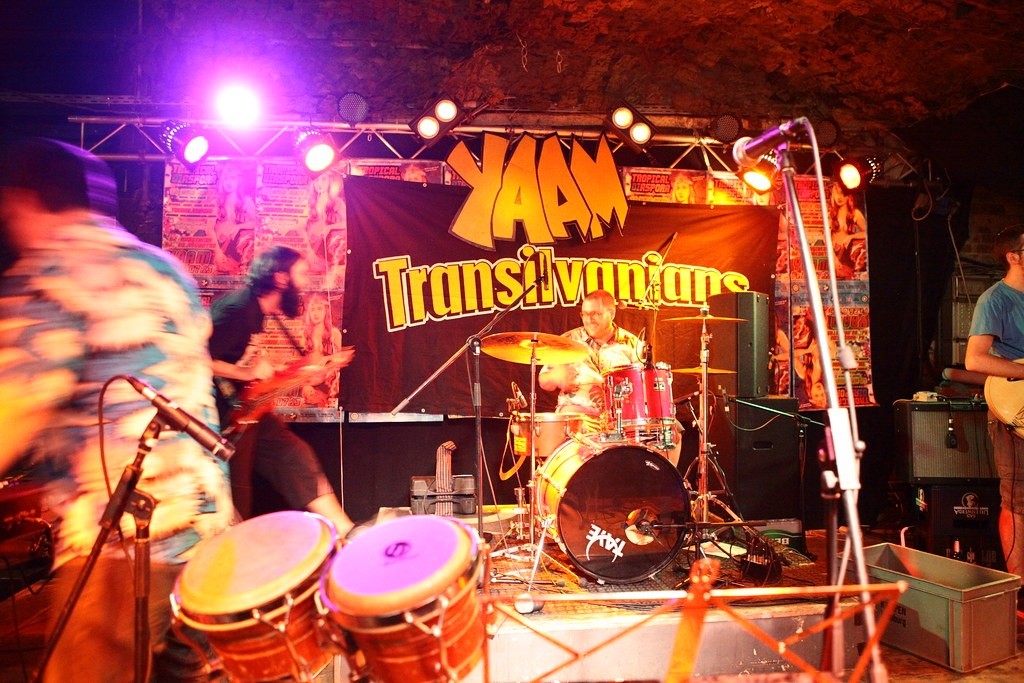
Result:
[963,222,1024,618]
[534,289,685,466]
[0,134,236,683]
[206,244,359,542]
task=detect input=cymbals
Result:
[480,332,591,365]
[671,366,738,378]
[661,314,749,324]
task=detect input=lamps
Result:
[407,93,467,150]
[712,111,742,143]
[604,97,659,154]
[296,125,335,173]
[838,153,884,197]
[157,119,210,167]
[337,92,371,128]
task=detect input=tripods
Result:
[389,252,564,587]
[767,149,888,682]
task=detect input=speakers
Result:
[706,395,798,519]
[706,290,768,398]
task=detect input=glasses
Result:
[579,309,611,318]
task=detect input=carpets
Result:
[479,533,862,618]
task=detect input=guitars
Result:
[983,356,1024,440]
[228,344,354,428]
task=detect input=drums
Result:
[514,412,584,458]
[316,513,486,683]
[602,361,675,429]
[169,511,337,683]
[536,432,692,584]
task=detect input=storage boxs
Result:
[410,474,477,515]
[750,519,803,552]
[836,542,1024,674]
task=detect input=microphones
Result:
[732,120,803,168]
